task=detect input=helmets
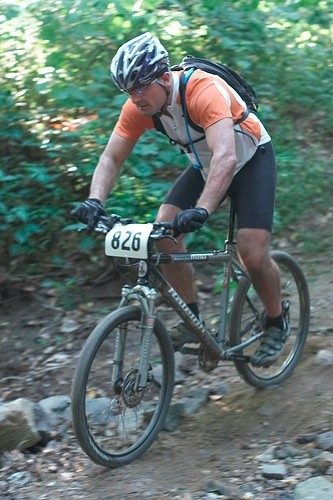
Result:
[109,32,170,92]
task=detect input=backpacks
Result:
[151,55,259,139]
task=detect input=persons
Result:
[71,32,291,368]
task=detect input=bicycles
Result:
[70,187,309,470]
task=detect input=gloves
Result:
[75,197,109,234]
[170,207,210,238]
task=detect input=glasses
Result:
[127,78,158,96]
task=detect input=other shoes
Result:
[250,314,291,368]
[166,319,207,352]
